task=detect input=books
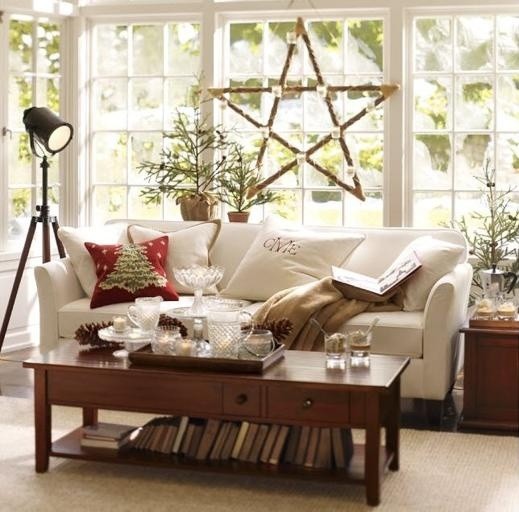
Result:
[331,251,421,297]
[80,415,354,469]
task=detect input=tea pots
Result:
[477,263,517,299]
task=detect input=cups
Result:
[495,296,517,321]
[112,316,127,333]
[150,305,275,361]
[348,330,372,370]
[323,331,346,371]
[474,297,495,321]
[127,297,162,330]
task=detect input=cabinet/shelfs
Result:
[459,326,519,431]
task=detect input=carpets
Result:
[0,394,519,512]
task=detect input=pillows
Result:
[59,221,135,297]
[83,235,179,311]
[221,222,366,301]
[382,235,469,312]
[128,218,222,297]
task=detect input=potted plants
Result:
[135,68,281,222]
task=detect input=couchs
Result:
[34,221,473,426]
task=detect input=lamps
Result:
[22,106,74,156]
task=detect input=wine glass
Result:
[171,265,225,317]
